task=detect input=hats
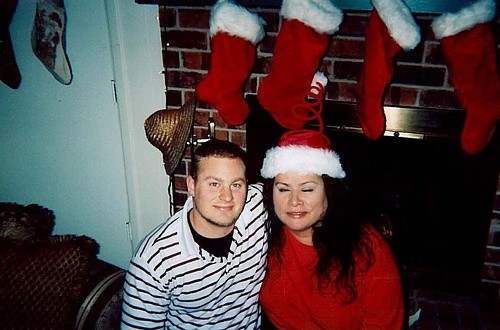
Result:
[144,96,198,176]
[259,130,347,179]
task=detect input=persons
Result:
[259,128,404,330]
[121,140,393,330]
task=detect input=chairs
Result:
[75,270,125,330]
